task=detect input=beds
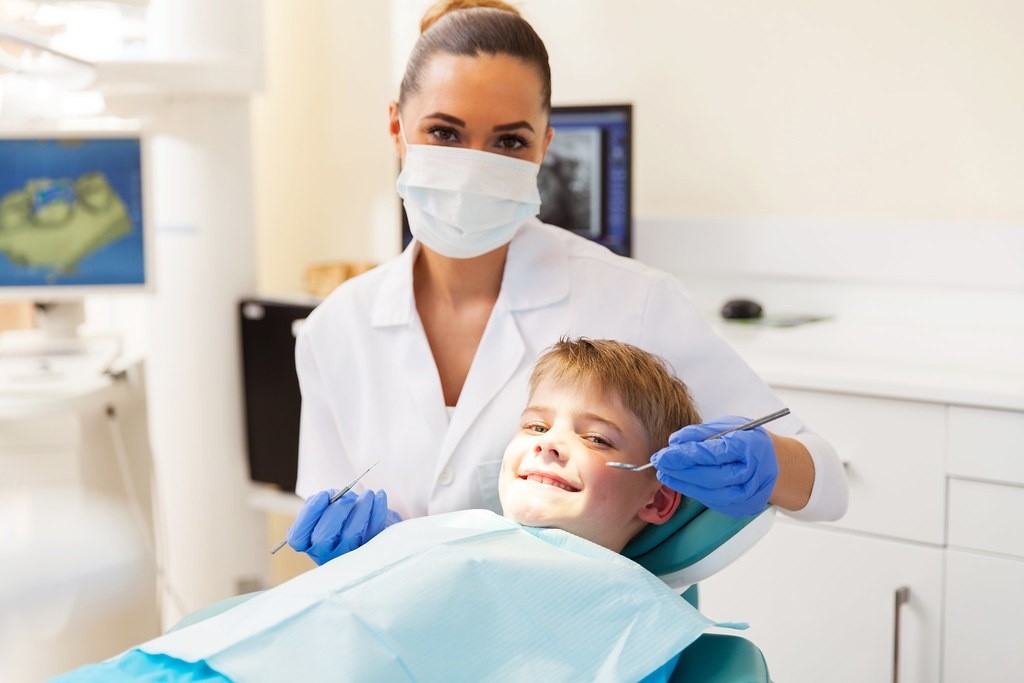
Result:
[34,463,771,683]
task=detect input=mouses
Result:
[722,300,762,320]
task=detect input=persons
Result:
[52,339,703,681]
[283,0,849,568]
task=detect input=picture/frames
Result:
[401,104,637,262]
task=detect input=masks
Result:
[396,111,542,259]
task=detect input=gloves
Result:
[285,489,404,567]
[650,416,778,518]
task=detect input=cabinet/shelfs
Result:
[695,310,1024,683]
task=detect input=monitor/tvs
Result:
[0,126,147,333]
[402,104,633,259]
